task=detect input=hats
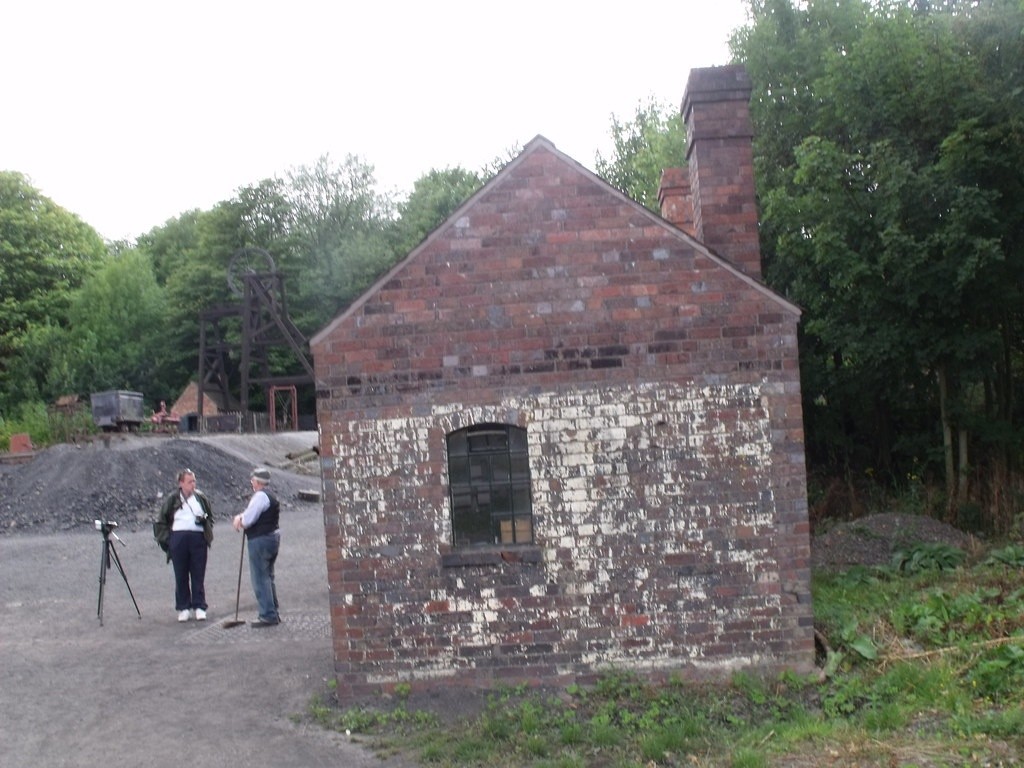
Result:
[249,468,271,482]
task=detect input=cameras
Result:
[94,520,118,533]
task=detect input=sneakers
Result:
[177,609,193,622]
[195,608,207,620]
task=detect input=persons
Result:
[152,467,216,622]
[233,466,282,629]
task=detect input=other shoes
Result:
[249,617,273,628]
